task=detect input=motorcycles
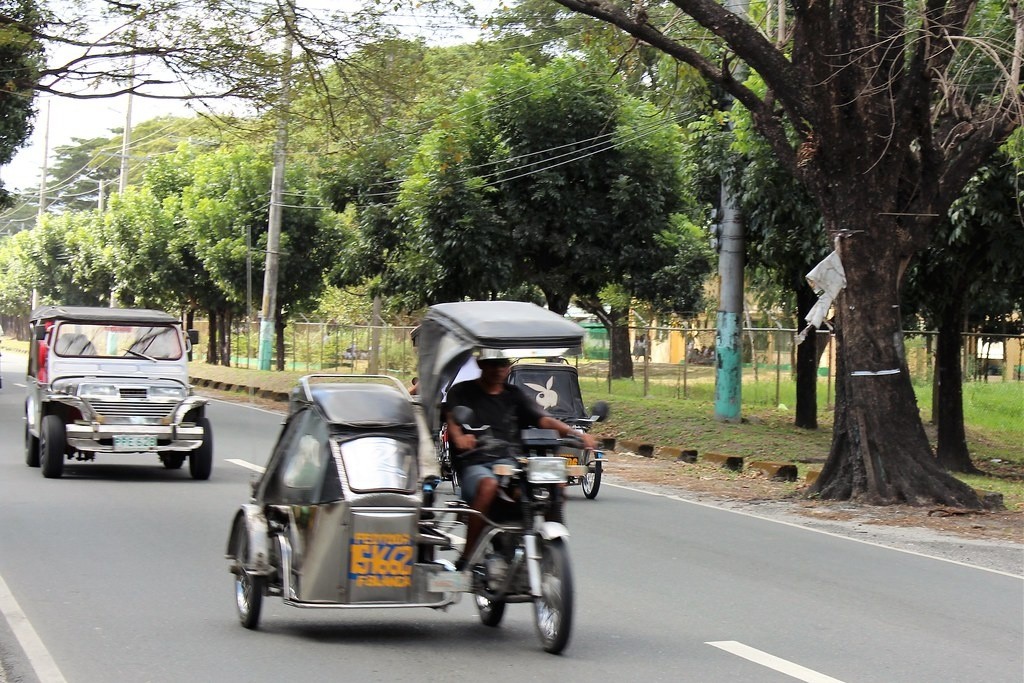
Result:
[225,302,617,654]
[411,324,609,498]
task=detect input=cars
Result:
[23,306,212,480]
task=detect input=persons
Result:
[442,360,598,574]
[407,376,421,395]
